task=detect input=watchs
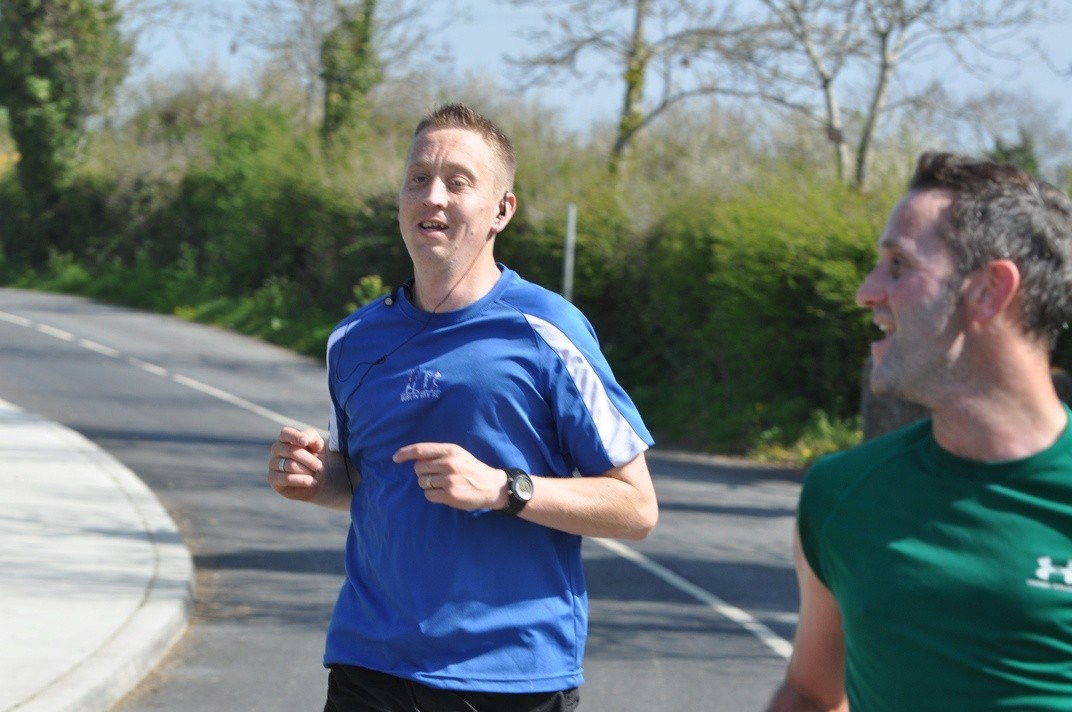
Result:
[503,468,535,515]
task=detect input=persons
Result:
[267,103,660,712]
[766,147,1072,712]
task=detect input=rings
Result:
[424,475,432,490]
[278,458,285,472]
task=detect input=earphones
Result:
[384,284,410,305]
[500,191,510,215]
